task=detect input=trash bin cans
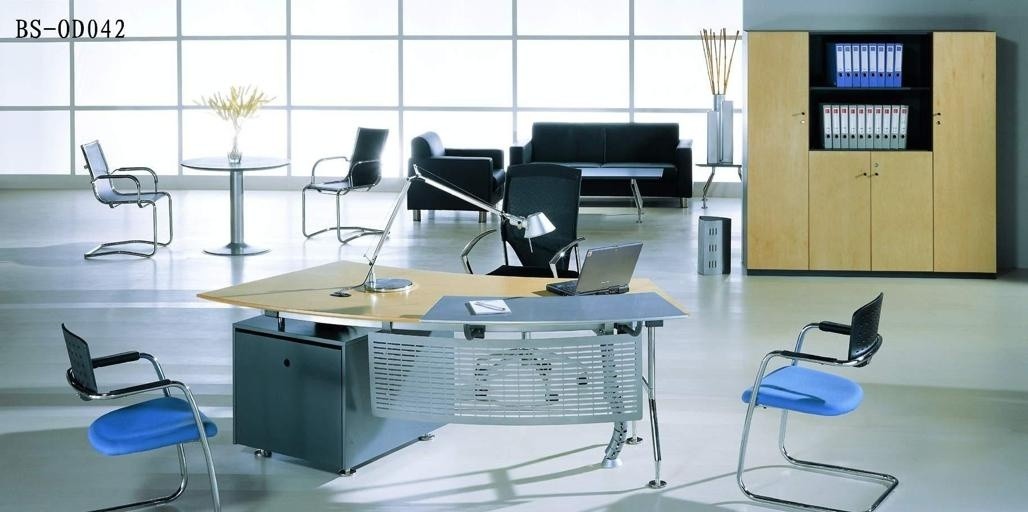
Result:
[698,216,731,275]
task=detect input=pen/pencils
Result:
[477,301,505,311]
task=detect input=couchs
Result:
[509,123,693,208]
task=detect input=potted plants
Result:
[192,84,278,164]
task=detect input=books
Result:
[469,299,511,316]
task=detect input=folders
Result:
[834,43,904,90]
[822,104,909,149]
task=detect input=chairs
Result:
[62,322,222,512]
[302,128,390,243]
[409,132,508,223]
[461,163,590,404]
[80,140,173,257]
[737,292,898,512]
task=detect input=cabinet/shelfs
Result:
[747,30,997,273]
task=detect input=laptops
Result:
[546,243,643,296]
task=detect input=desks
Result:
[196,260,690,489]
[696,164,742,209]
[180,157,291,255]
